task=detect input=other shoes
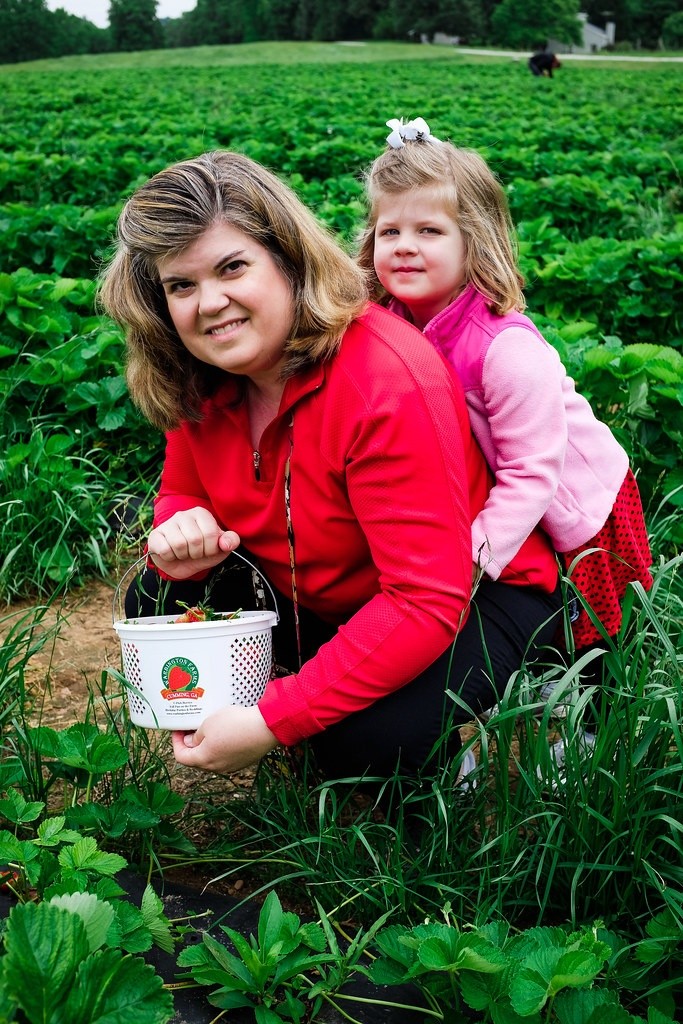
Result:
[451,742,476,796]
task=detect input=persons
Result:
[100,149,561,796]
[355,116,655,790]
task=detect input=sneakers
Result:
[477,671,572,724]
[535,727,628,789]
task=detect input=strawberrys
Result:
[175,606,241,624]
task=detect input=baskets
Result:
[113,546,282,732]
